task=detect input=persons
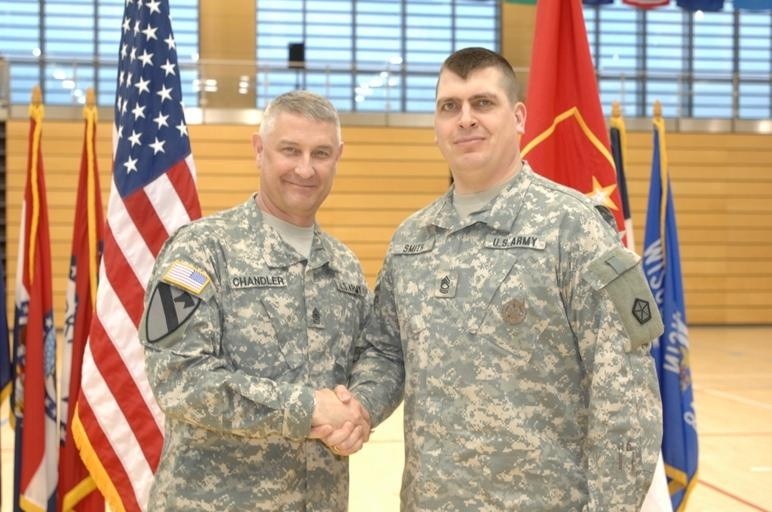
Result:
[139,89,382,512]
[306,47,664,512]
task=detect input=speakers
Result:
[288,44,305,68]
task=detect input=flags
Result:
[642,120,700,512]
[61,106,100,438]
[16,102,54,511]
[522,2,625,244]
[60,0,198,512]
[609,117,635,253]
[1,262,12,406]
